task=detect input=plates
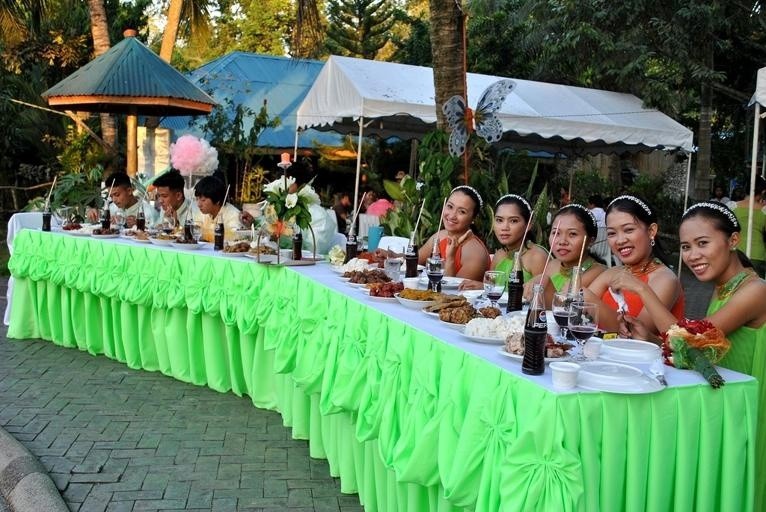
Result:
[334,260,666,394]
[60,225,281,261]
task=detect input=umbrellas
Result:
[40,29,216,177]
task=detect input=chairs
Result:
[376,236,410,272]
[5,212,63,324]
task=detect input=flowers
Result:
[659,316,730,390]
[250,153,321,239]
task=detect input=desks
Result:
[6,228,766,512]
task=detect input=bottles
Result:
[345,224,584,377]
[42,198,304,260]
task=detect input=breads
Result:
[504,331,554,354]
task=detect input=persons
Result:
[86,172,153,228]
[417,175,766,382]
[146,172,192,231]
[164,176,241,241]
[240,162,406,255]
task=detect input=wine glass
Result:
[386,245,599,363]
